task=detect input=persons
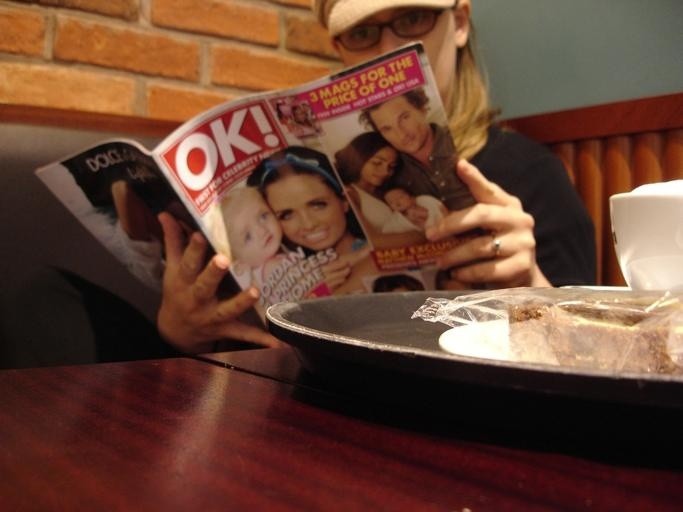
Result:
[148,2,598,358]
[208,188,294,291]
[379,189,447,236]
[360,86,475,210]
[246,143,378,293]
[334,131,428,251]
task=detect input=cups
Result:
[610,178,682,295]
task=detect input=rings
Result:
[491,236,501,258]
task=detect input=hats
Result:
[313,0,457,38]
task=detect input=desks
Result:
[0,349,682,512]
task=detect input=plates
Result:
[440,316,519,361]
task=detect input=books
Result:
[35,42,479,330]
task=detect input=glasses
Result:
[333,7,447,52]
